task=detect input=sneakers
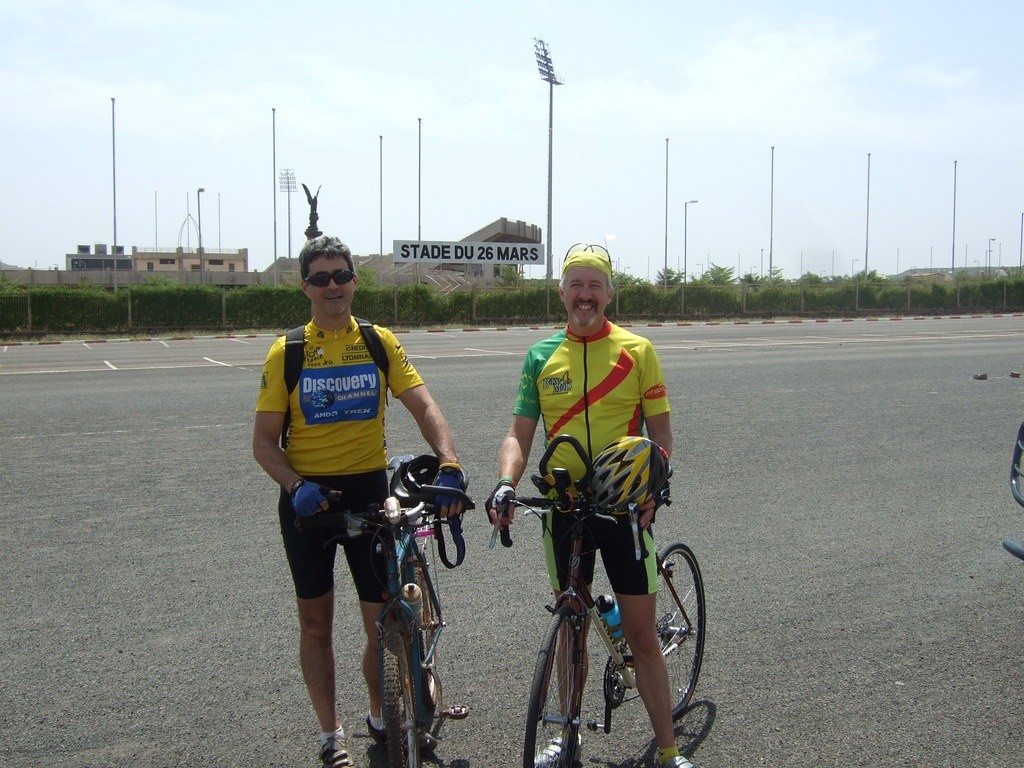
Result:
[365,714,438,752]
[656,755,696,768]
[534,733,582,768]
[318,738,356,768]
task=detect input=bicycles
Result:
[294,500,476,768]
[489,434,706,768]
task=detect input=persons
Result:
[485,243,695,768]
[253,236,463,766]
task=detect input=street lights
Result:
[761,249,764,279]
[852,260,859,277]
[198,188,205,282]
[418,118,421,242]
[665,138,669,294]
[952,160,957,274]
[770,146,775,277]
[1020,213,1024,275]
[271,108,276,286]
[110,97,118,292]
[865,153,871,282]
[989,239,996,280]
[379,135,383,288]
[532,38,563,281]
[697,264,703,278]
[685,200,699,284]
[986,250,993,273]
[973,261,979,272]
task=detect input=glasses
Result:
[305,269,355,287]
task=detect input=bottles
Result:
[401,582,423,618]
[595,594,624,642]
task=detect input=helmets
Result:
[389,454,470,509]
[590,435,669,514]
